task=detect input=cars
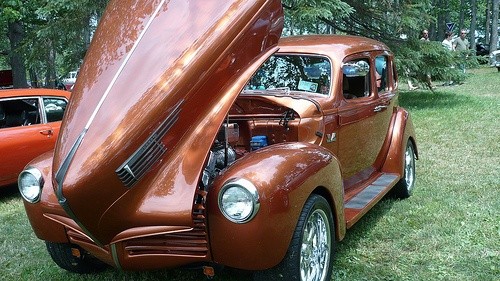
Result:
[63,71,78,90]
[17,0,420,281]
[0,88,72,188]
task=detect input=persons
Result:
[418,27,499,56]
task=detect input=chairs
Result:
[5,109,26,127]
[318,72,356,100]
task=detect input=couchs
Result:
[306,75,366,98]
[29,110,65,123]
